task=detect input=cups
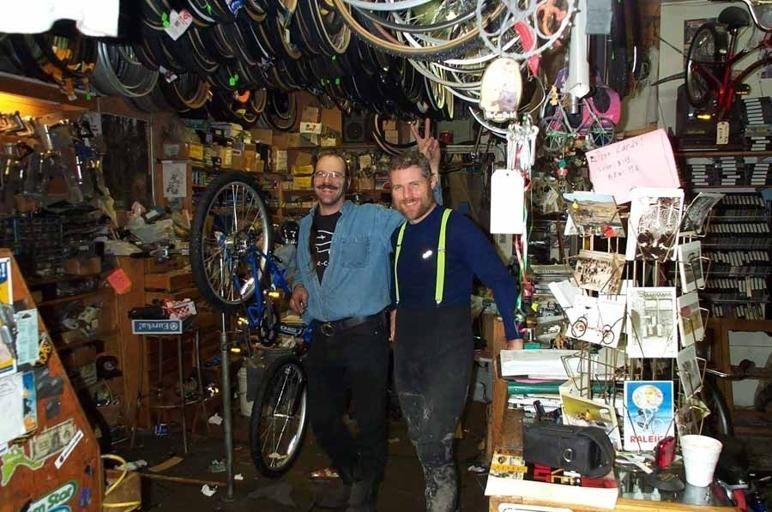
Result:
[680,434,722,487]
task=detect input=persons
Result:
[387,150,524,512]
[288,118,441,512]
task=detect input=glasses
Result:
[315,169,346,181]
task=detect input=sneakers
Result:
[335,480,379,512]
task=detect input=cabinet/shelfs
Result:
[0,71,772,512]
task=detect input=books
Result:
[483,446,619,509]
[688,97,772,321]
[496,346,586,415]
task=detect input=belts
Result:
[312,316,376,336]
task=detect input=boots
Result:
[424,462,458,512]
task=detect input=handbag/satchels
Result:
[523,423,615,479]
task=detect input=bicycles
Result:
[186,169,317,478]
[685,0,770,120]
